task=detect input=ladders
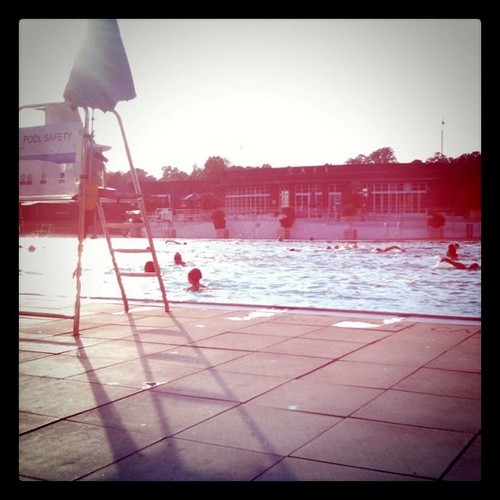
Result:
[20,102,168,335]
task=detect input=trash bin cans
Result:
[465,222,474,235]
[128,218,144,238]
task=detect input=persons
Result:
[276,237,479,270]
[146,252,208,293]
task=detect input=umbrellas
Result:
[63,20,137,113]
